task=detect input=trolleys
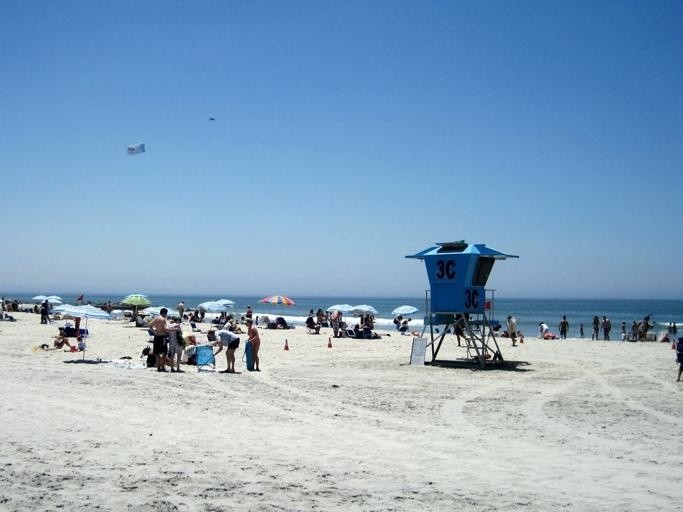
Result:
[47,309,58,325]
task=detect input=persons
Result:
[0,296,53,324]
[454,314,470,346]
[489,313,683,381]
[137,301,291,373]
[306,309,411,340]
[76,293,137,314]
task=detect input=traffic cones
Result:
[670,339,678,352]
[327,336,333,348]
[283,339,290,351]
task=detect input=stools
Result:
[306,327,315,333]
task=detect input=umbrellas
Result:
[325,303,419,339]
[120,293,152,314]
[198,298,235,331]
[257,295,296,320]
[31,294,113,363]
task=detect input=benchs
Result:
[136,315,150,327]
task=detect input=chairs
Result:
[34,306,65,314]
[196,346,215,371]
[59,327,89,337]
